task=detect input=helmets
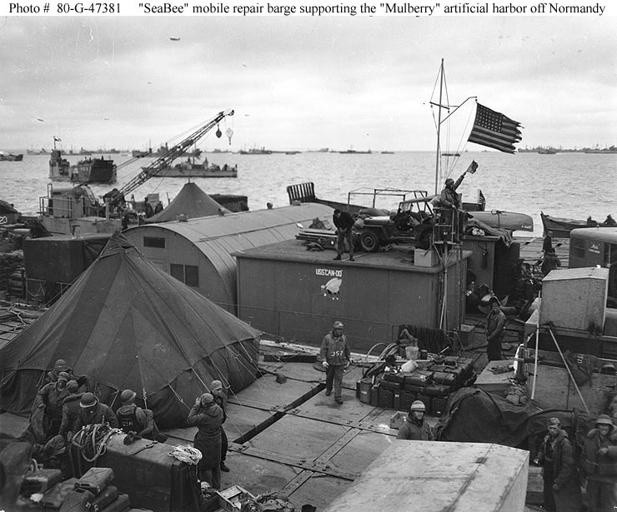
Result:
[54,359,79,393]
[119,389,137,404]
[332,321,345,333]
[594,414,613,428]
[410,400,425,412]
[79,392,98,408]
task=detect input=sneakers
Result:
[325,389,343,404]
[350,254,355,261]
[333,255,341,260]
[220,462,229,473]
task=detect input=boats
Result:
[538,149,556,154]
[26,147,122,156]
[539,211,616,237]
[239,148,272,155]
[0,151,24,162]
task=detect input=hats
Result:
[445,178,454,186]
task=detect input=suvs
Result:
[355,195,466,251]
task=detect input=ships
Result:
[141,166,238,179]
[0,58,617,510]
[131,142,201,158]
[48,134,117,185]
[338,149,372,154]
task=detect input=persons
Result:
[464,278,491,314]
[534,416,585,512]
[320,322,353,403]
[331,208,357,262]
[605,214,616,225]
[483,300,507,361]
[19,356,232,493]
[540,246,562,277]
[579,413,616,512]
[96,193,165,230]
[396,399,436,440]
[541,230,554,252]
[440,172,467,242]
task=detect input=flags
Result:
[467,160,479,174]
[467,103,527,153]
